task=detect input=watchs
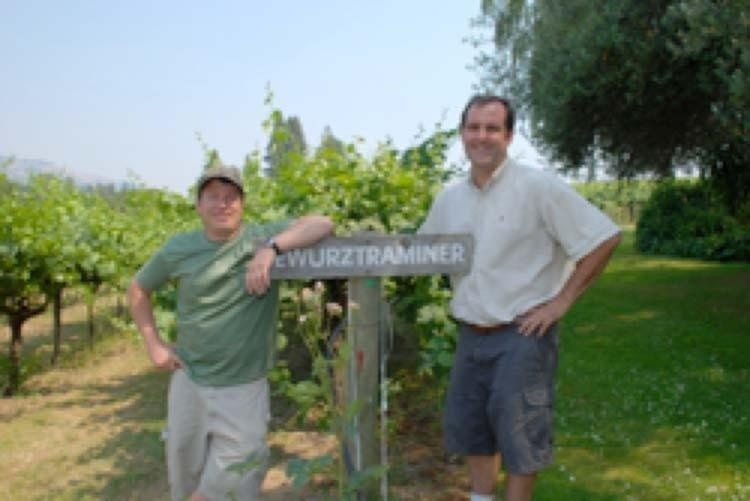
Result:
[261,237,285,257]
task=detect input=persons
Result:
[411,92,624,500]
[122,165,335,500]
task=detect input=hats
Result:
[198,164,243,194]
